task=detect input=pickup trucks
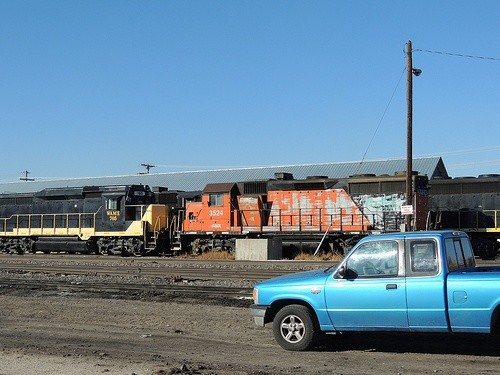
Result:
[251,229,500,351]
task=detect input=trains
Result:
[1,169,499,257]
